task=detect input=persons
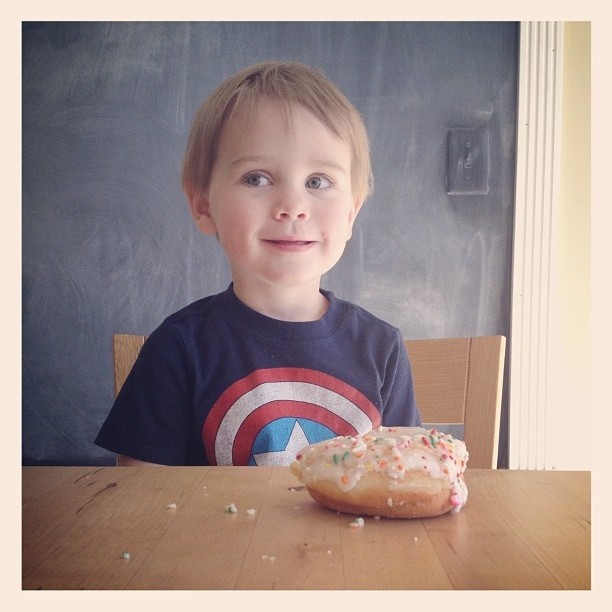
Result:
[94,60,422,467]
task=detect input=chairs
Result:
[115,333,506,470]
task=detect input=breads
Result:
[291,428,470,518]
